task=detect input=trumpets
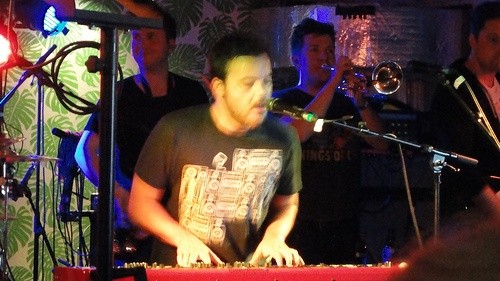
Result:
[321,59,404,95]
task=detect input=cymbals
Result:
[0,149,19,159]
[0,137,27,147]
[5,155,63,162]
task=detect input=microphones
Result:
[265,97,316,122]
[407,60,457,77]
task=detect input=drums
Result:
[0,178,18,199]
[0,149,19,179]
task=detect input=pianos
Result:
[52,255,417,281]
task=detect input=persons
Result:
[128,30,304,267]
[428,2,500,220]
[75,4,209,267]
[395,213,500,281]
[272,18,388,265]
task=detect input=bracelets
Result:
[357,98,369,110]
